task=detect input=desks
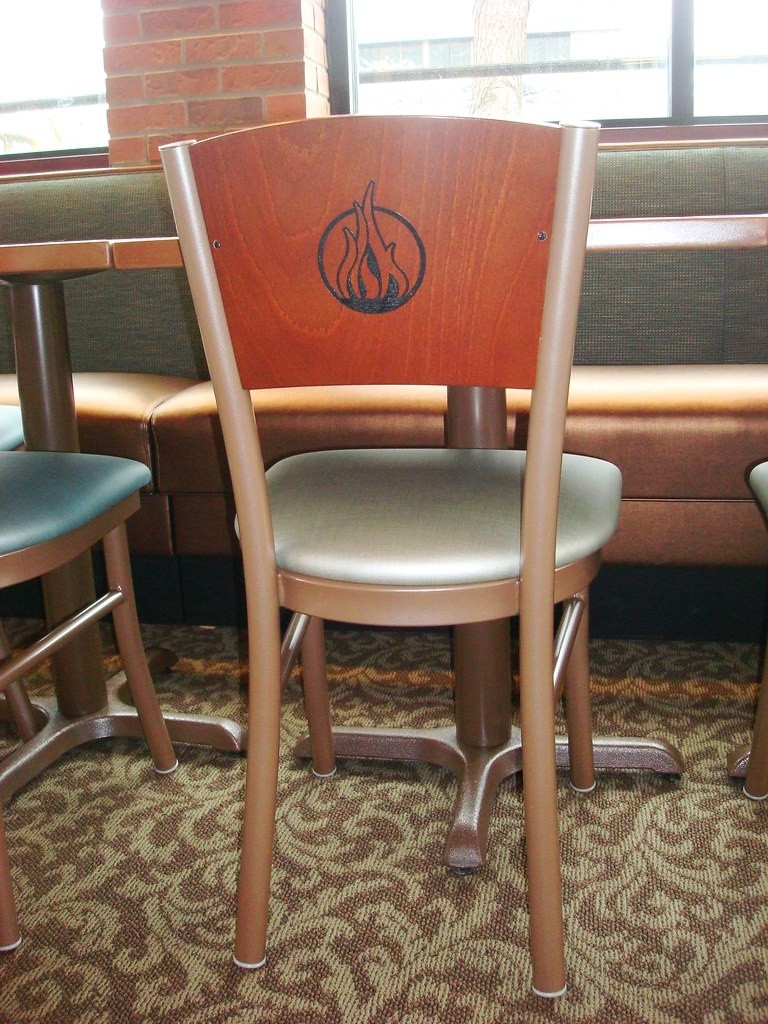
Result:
[111,212,768,871]
[0,238,247,814]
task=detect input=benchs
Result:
[0,142,768,569]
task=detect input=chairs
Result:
[0,448,179,953]
[152,113,624,997]
